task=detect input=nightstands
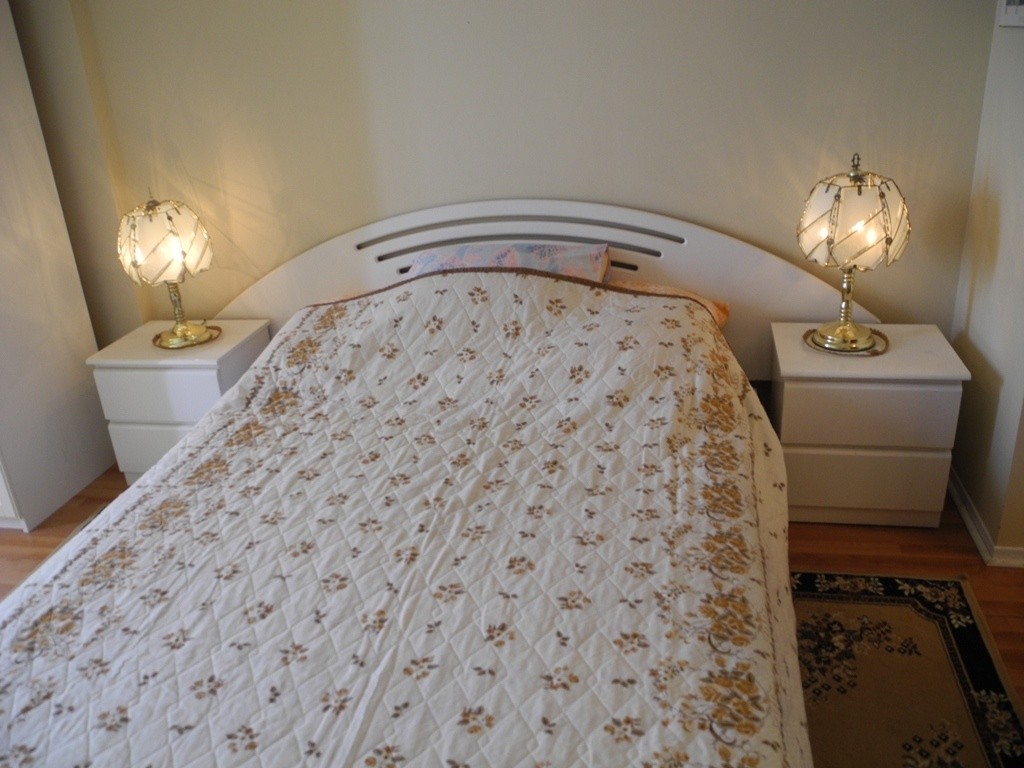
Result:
[85,320,271,487]
[769,322,971,529]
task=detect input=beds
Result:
[0,202,884,768]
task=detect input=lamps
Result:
[795,153,911,356]
[116,188,221,349]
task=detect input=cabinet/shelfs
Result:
[1,0,115,535]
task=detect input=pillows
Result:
[405,243,610,285]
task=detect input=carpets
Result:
[789,570,1024,768]
